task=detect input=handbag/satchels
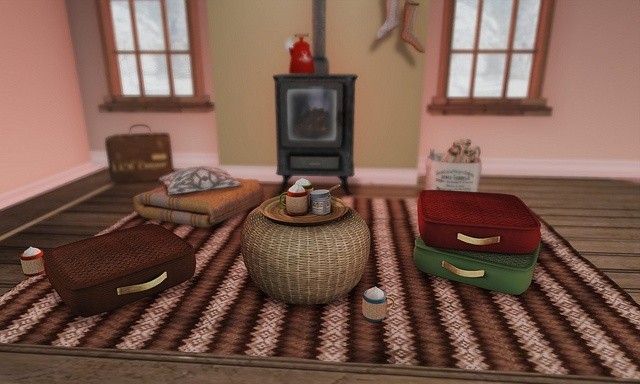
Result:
[44,222,196,316]
[417,189,542,253]
[106,125,172,184]
[414,235,540,296]
